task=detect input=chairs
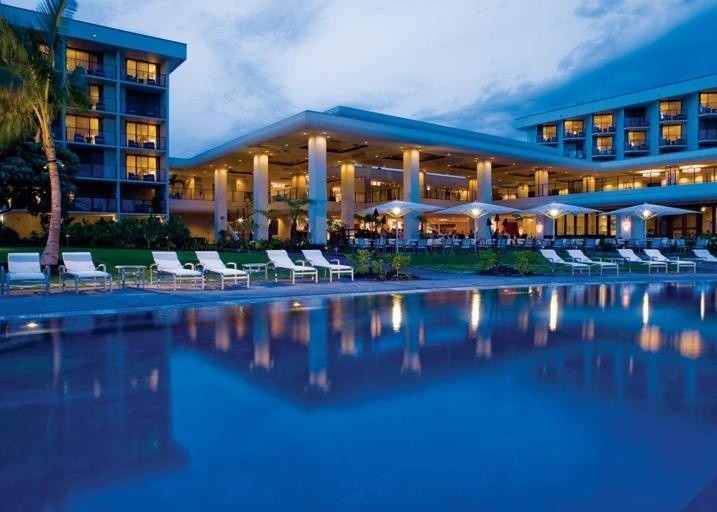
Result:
[4,251,113,298]
[149,250,251,292]
[538,246,717,277]
[265,248,356,285]
[358,236,712,256]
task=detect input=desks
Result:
[116,264,148,291]
[241,264,269,280]
